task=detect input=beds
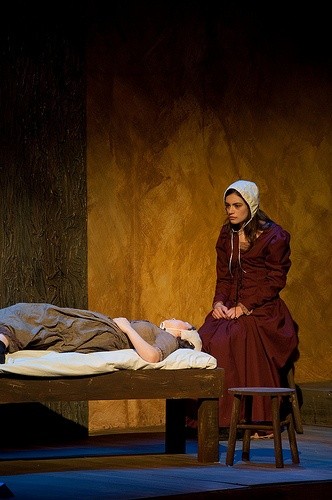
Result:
[1,349,225,462]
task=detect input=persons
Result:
[186,179,300,440]
[0,303,203,363]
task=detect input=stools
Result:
[225,388,300,468]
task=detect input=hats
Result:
[223,180,259,223]
